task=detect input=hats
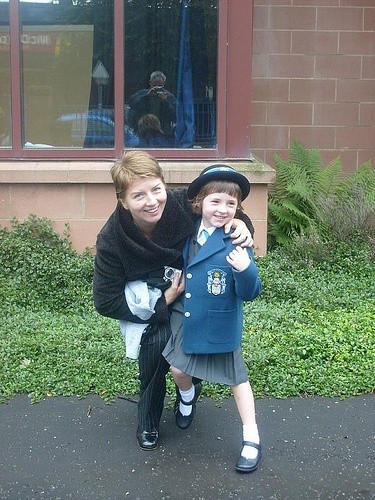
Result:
[187,164,250,202]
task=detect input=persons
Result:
[162,165,262,472]
[92,150,256,450]
[128,71,177,148]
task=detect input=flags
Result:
[177,5,194,147]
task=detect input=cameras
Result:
[162,266,182,282]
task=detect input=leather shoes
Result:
[176,393,196,430]
[235,441,263,471]
[136,428,160,451]
